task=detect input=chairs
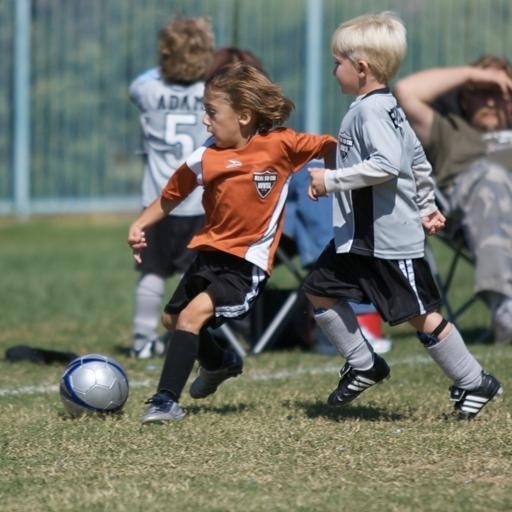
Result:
[423,225,495,336]
[253,241,311,355]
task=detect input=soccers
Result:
[60,354,129,420]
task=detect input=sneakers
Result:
[327,353,390,406]
[443,371,500,420]
[191,355,242,399]
[132,333,165,360]
[143,399,186,422]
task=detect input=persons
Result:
[126,60,339,424]
[206,46,395,357]
[297,7,507,419]
[395,55,512,346]
[127,9,216,360]
